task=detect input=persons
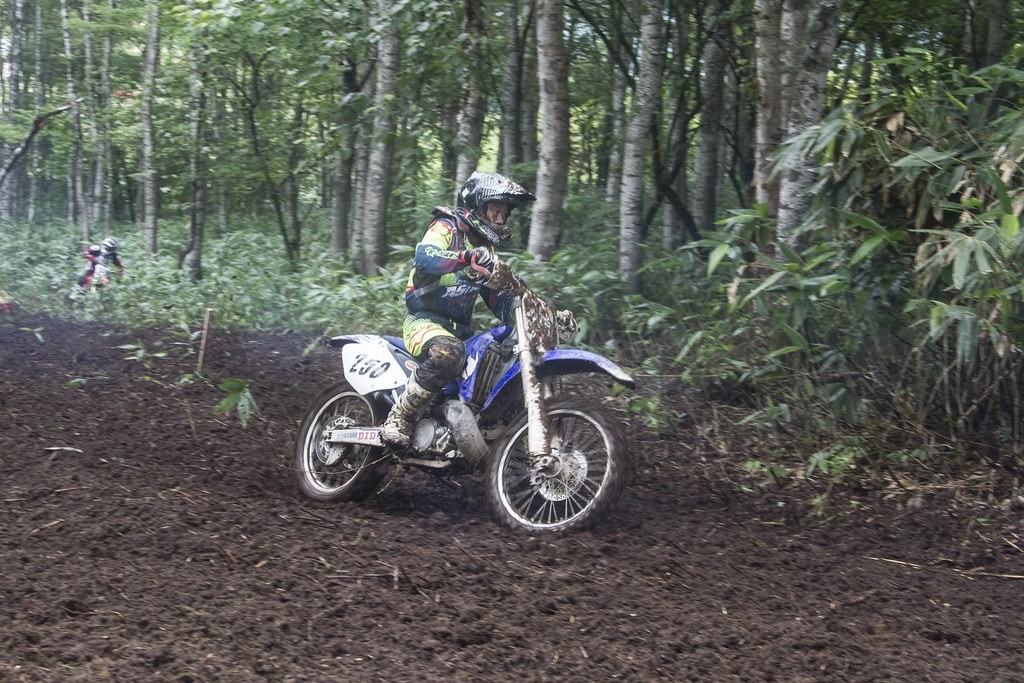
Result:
[376,171,579,450]
[65,235,126,307]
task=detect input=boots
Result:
[383,369,441,451]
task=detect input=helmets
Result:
[101,238,119,256]
[457,171,536,248]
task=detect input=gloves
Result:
[558,309,578,339]
[464,246,494,270]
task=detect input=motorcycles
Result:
[85,244,120,299]
[293,251,636,538]
